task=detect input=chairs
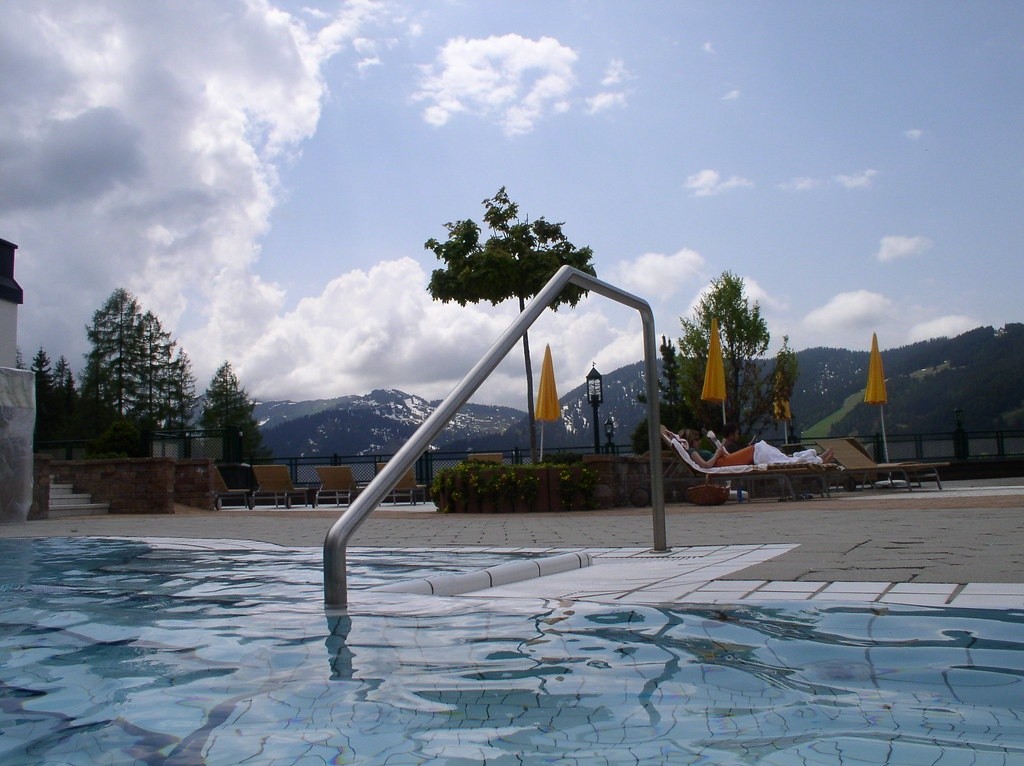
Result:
[313,467,373,507]
[661,425,952,504]
[375,463,426,507]
[213,467,251,510]
[251,465,309,509]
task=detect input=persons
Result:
[677,422,834,469]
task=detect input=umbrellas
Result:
[772,347,791,446]
[701,318,727,425]
[534,343,561,462]
[864,331,907,489]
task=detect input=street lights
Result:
[587,366,604,452]
[605,418,616,452]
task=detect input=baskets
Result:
[687,474,730,505]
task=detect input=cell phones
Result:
[750,436,756,444]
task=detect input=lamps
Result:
[787,415,797,430]
[604,419,614,436]
[952,409,965,426]
